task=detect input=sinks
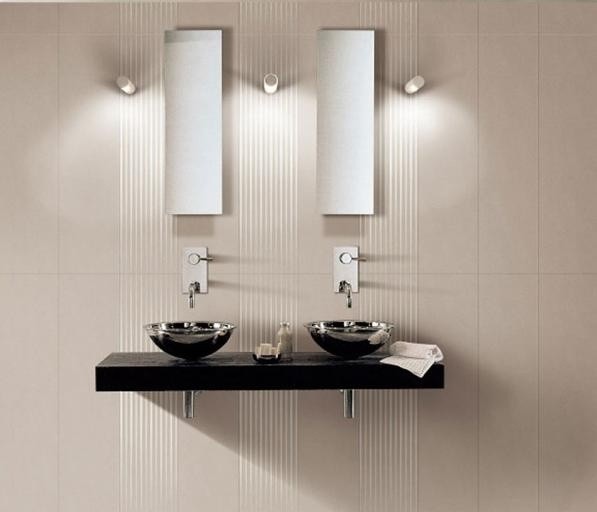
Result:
[302,318,395,358]
[143,320,237,360]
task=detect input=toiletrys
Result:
[278,322,294,358]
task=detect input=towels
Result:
[377,340,445,377]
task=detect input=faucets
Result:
[187,282,199,308]
[335,279,351,308]
